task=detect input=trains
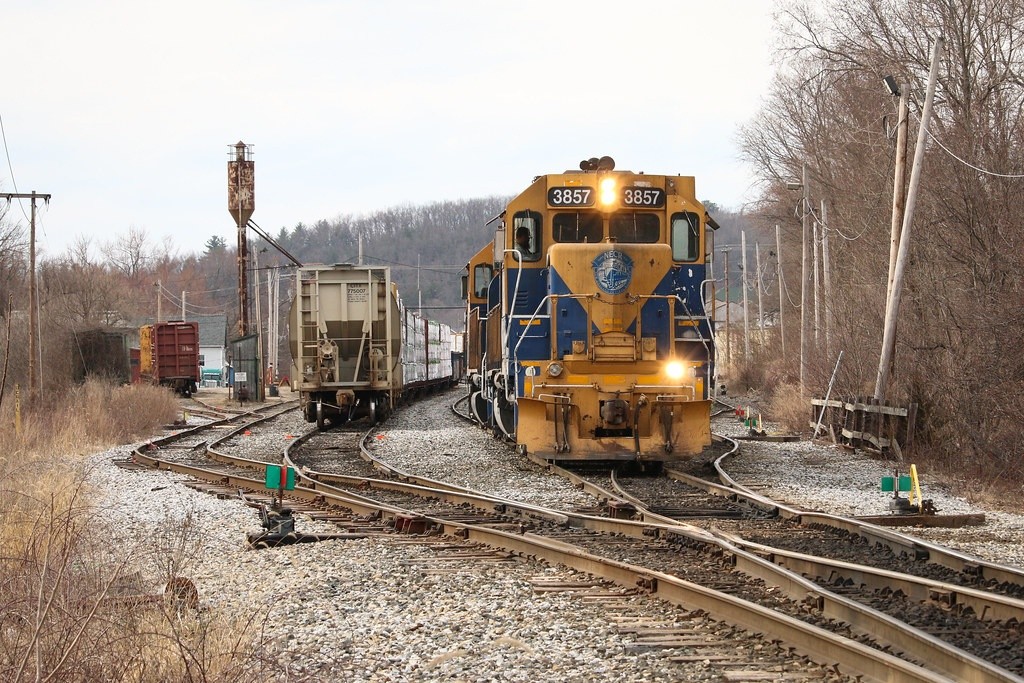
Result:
[291,261,464,431]
[453,156,721,477]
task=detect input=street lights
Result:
[880,72,900,375]
[784,165,819,411]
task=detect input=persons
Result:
[514,227,540,261]
